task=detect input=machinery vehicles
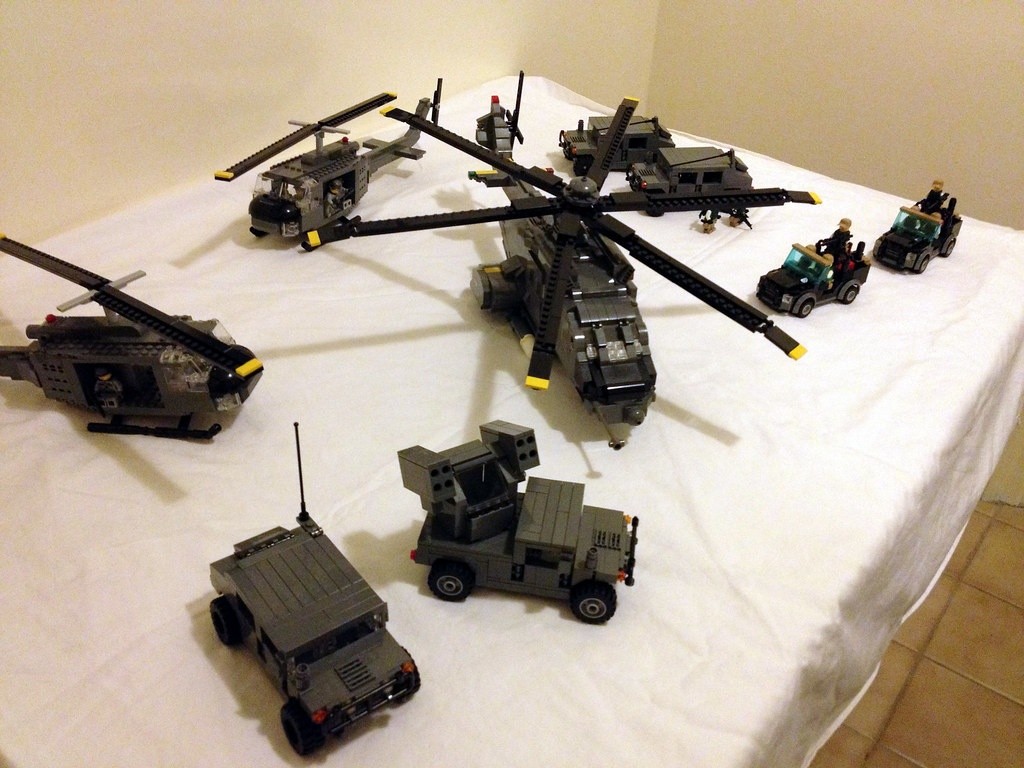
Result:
[397,420,640,626]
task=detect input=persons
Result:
[920,179,945,215]
[292,186,304,201]
[822,218,852,267]
[899,206,920,230]
[94,366,123,396]
[921,211,942,240]
[325,180,349,217]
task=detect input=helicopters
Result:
[0,231,264,440]
[214,77,442,252]
[306,71,822,450]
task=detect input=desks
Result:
[0,74,1021,768]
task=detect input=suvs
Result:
[209,423,421,755]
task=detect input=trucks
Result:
[625,147,755,217]
[558,116,676,177]
